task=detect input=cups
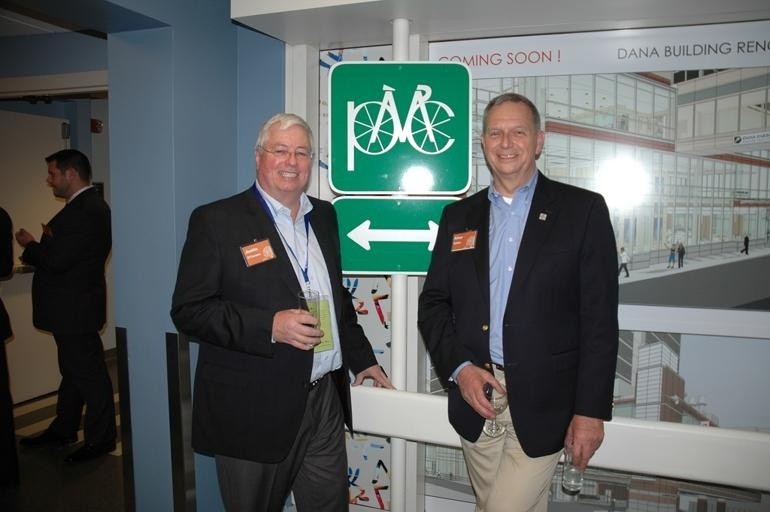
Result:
[298,289,321,348]
[561,437,585,499]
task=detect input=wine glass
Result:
[481,380,508,438]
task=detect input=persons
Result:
[677,244,685,268]
[667,243,676,268]
[741,234,749,255]
[0,207,21,503]
[170,112,397,511]
[417,92,620,512]
[618,247,629,277]
[14,147,117,464]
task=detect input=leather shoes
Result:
[20,427,78,446]
[64,442,116,462]
